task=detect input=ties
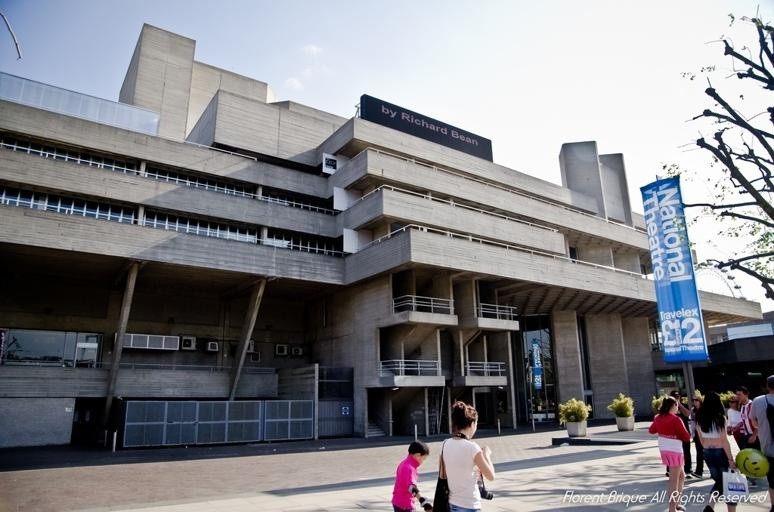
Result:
[742,407,749,435]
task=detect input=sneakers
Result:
[666,471,702,478]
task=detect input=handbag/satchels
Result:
[431,478,449,512]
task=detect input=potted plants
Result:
[558,396,594,439]
[605,393,639,434]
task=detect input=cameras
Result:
[481,489,493,500]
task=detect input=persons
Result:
[439,400,496,512]
[647,376,774,512]
[391,441,433,512]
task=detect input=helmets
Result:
[692,399,698,402]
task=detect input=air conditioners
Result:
[114,332,179,351]
[290,346,304,356]
[275,344,288,356]
[178,334,196,352]
[206,342,218,352]
[247,339,254,353]
